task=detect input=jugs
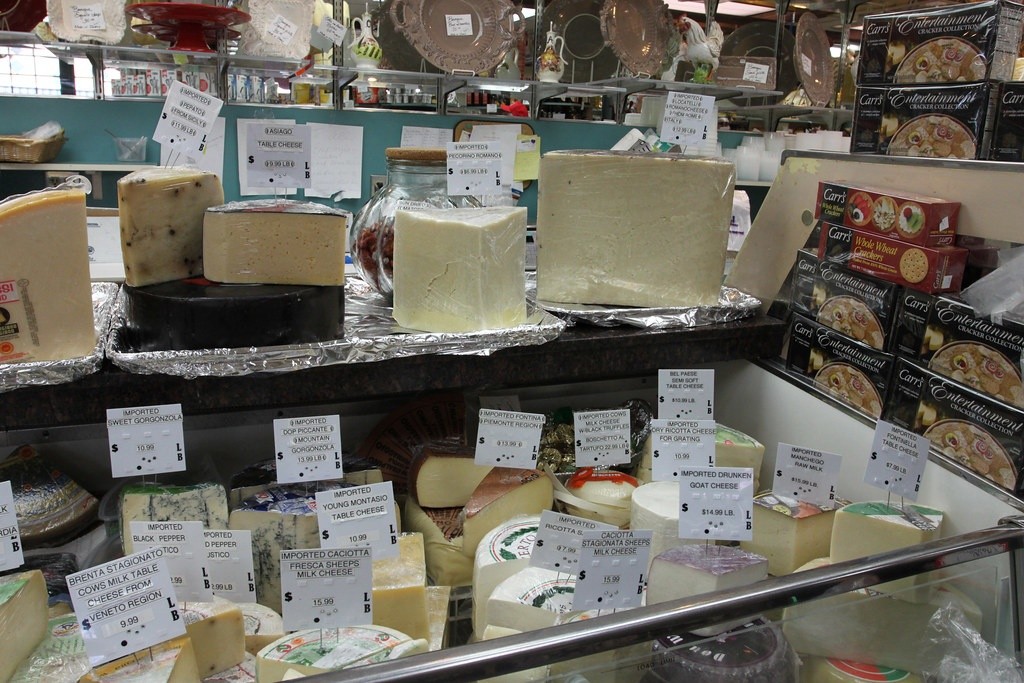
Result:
[347,13,383,70]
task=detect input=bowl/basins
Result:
[723,131,851,181]
[113,137,147,161]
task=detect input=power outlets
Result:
[46,171,79,188]
[370,175,386,198]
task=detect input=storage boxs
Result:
[784,179,1023,502]
[849,0,1023,163]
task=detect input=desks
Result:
[734,180,773,186]
[0,162,163,172]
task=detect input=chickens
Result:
[678,14,724,81]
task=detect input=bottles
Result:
[349,147,483,305]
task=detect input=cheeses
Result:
[0,147,984,683]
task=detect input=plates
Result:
[886,113,977,160]
[390,0,527,76]
[600,0,673,78]
[720,22,796,93]
[793,12,834,106]
[46,0,126,45]
[237,0,313,60]
[813,362,883,417]
[540,0,618,83]
[892,36,988,83]
[927,340,1023,406]
[816,294,885,350]
[923,418,1018,491]
